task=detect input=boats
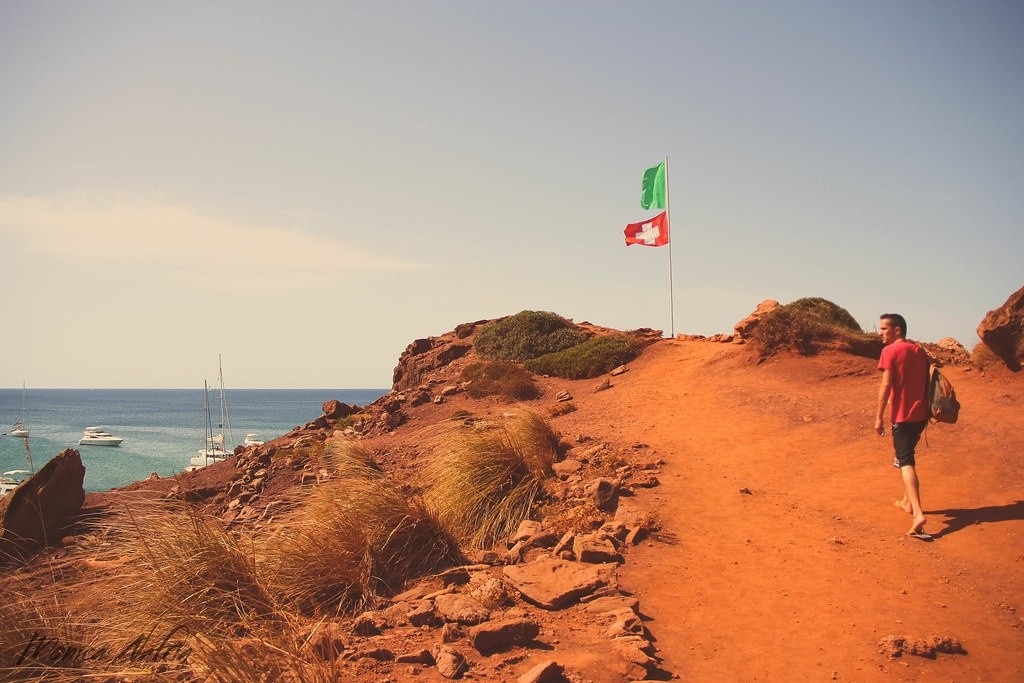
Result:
[78,426,124,446]
[244,433,264,446]
[0,470,31,496]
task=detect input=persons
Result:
[875,314,934,539]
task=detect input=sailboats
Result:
[183,352,236,473]
[5,379,31,438]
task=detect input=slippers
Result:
[910,527,932,539]
[895,500,913,515]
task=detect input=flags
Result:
[641,160,667,210]
[625,212,668,246]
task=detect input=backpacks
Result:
[916,341,960,424]
